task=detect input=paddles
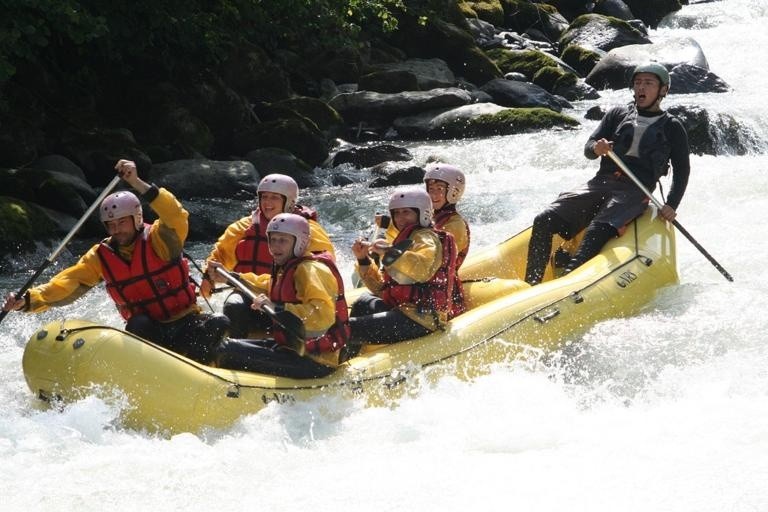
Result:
[215,266,303,341]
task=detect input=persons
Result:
[2,160,231,366]
[524,63,691,285]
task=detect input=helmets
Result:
[424,164,465,204]
[632,61,671,89]
[256,174,298,213]
[266,213,309,257]
[390,190,431,228]
[100,191,143,231]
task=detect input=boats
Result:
[21,193,679,442]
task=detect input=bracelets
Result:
[139,183,157,205]
